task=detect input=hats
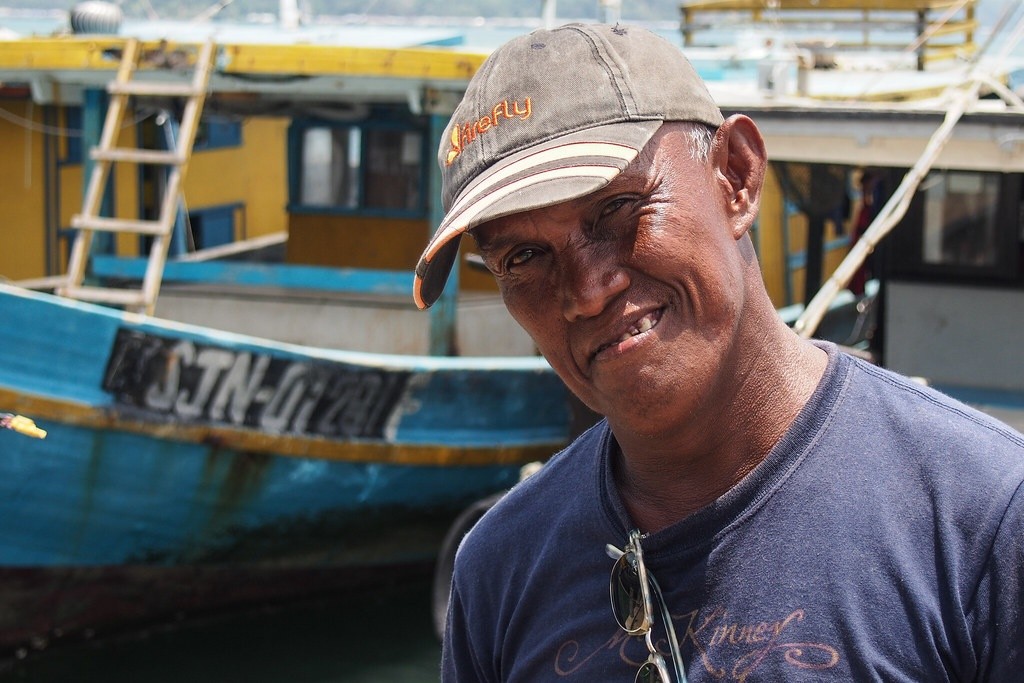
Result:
[411,21,727,314]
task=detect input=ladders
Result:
[54,37,217,318]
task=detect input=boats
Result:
[1,251,875,594]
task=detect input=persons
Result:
[413,21,1024,683]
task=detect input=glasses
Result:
[605,527,687,683]
